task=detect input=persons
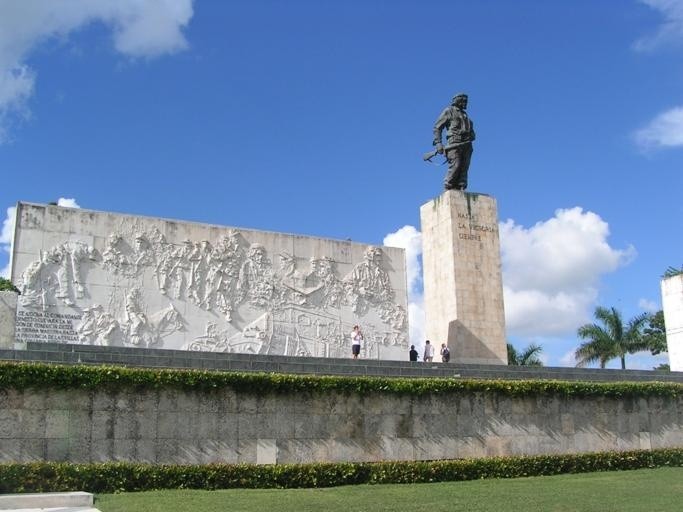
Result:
[423,340,435,362]
[432,94,474,190]
[440,344,450,362]
[410,345,418,361]
